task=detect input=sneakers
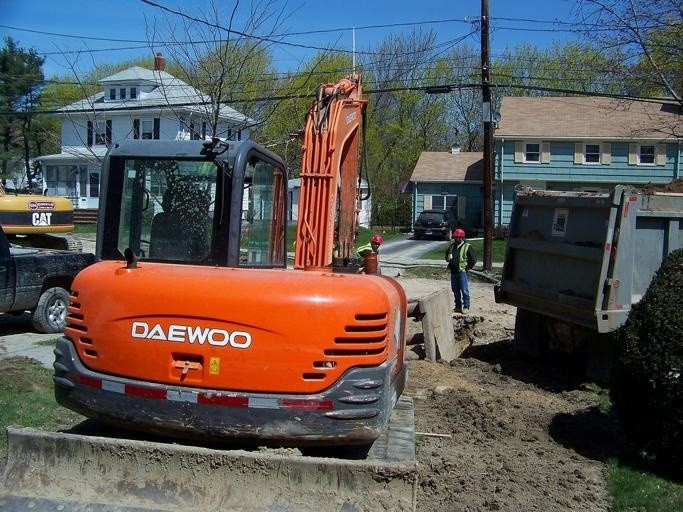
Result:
[462,308,470,314]
[453,308,462,313]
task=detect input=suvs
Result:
[414,210,461,240]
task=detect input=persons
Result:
[445,228,476,314]
[355,235,384,275]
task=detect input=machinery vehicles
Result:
[0,182,96,333]
[494,184,683,350]
[2,73,474,512]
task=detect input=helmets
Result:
[453,229,465,238]
[371,234,383,244]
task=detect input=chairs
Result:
[148,190,210,261]
